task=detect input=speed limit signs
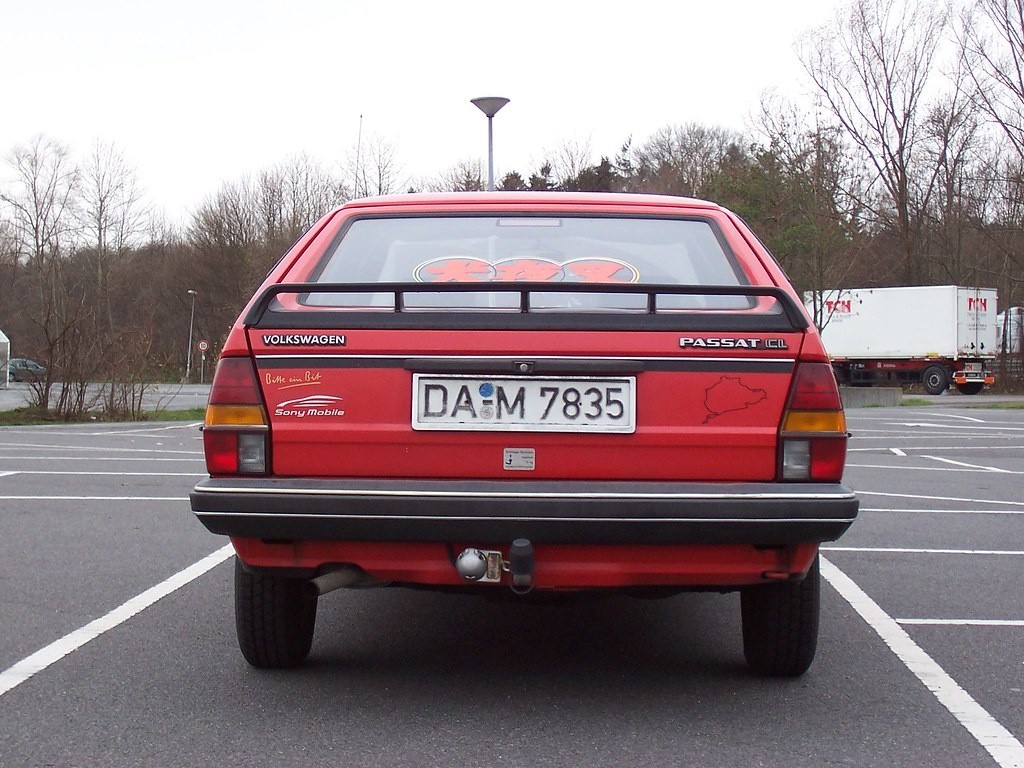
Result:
[197,340,209,352]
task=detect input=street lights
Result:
[472,96,508,195]
[185,290,198,376]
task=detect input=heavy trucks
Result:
[996,307,1022,354]
[803,285,999,395]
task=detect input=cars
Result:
[8,358,47,383]
[190,193,863,676]
